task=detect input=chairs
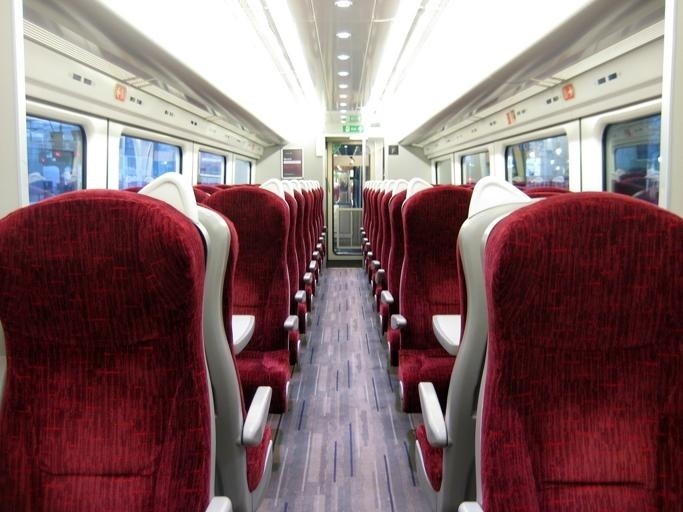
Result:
[359,180,682,512]
[0,179,327,512]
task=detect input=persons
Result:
[337,174,355,205]
